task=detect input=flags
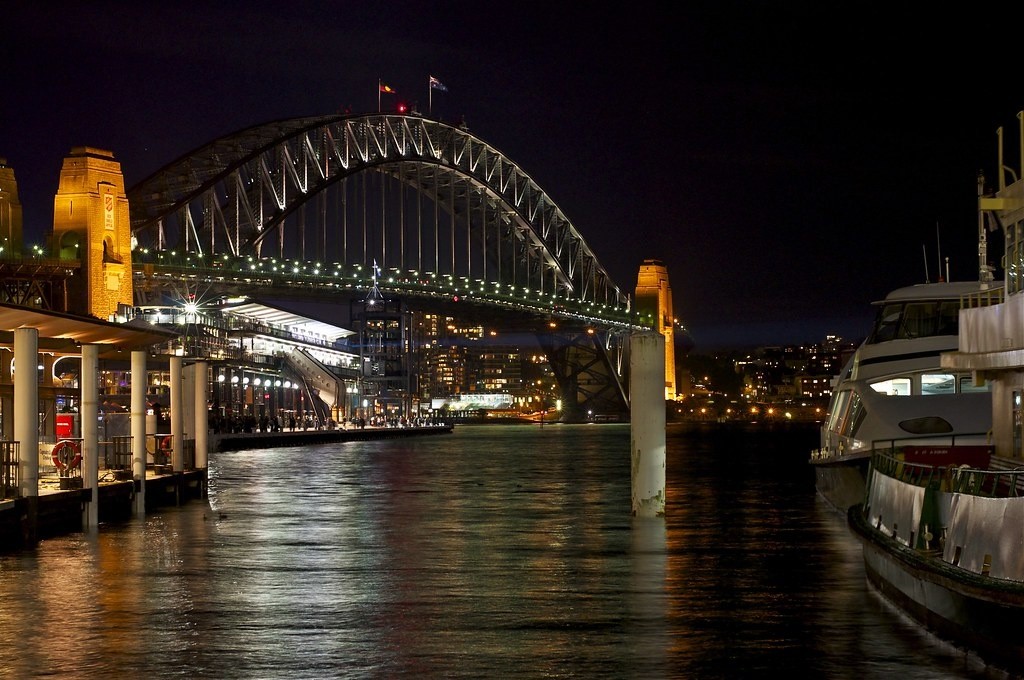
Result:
[379,79,396,93]
[429,76,448,92]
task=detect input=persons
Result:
[208,408,445,432]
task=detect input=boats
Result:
[845,280,1024,680]
[807,221,1005,520]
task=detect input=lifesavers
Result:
[162,436,171,457]
[51,441,80,470]
